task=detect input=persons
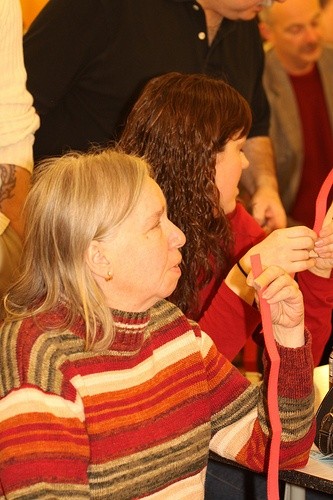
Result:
[111,71,333,373]
[262,0,333,231]
[0,2,40,329]
[22,0,285,237]
[0,148,317,500]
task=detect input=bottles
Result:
[313,352,333,457]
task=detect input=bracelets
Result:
[236,261,248,277]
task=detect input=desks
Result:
[207,365,333,500]
